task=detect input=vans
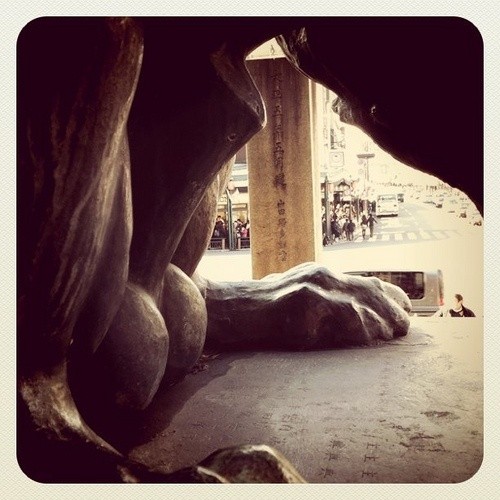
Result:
[376,192,399,217]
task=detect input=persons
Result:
[211,215,250,248]
[322,209,341,244]
[347,220,355,241]
[440,294,476,317]
[360,215,368,239]
[367,214,377,238]
[342,219,350,241]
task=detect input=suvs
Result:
[340,248,443,317]
